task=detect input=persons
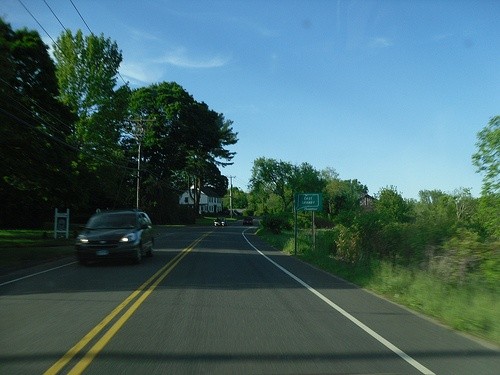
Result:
[215,217,226,223]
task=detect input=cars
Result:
[75,210,154,266]
[243,217,253,226]
[214,217,228,227]
